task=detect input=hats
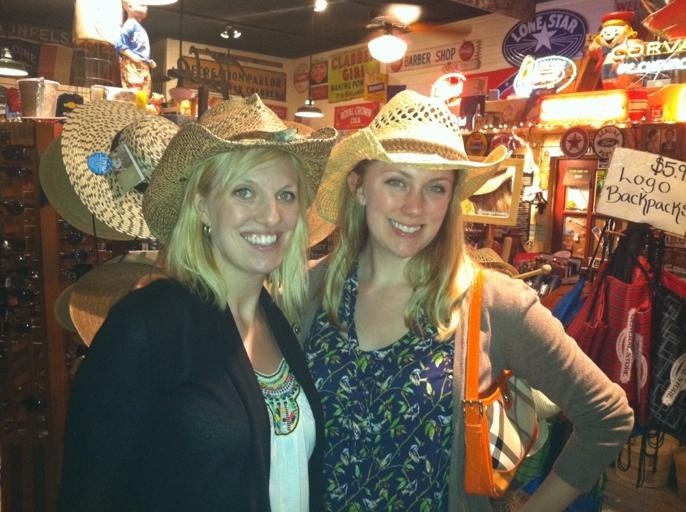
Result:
[62,100,182,239]
[40,137,136,240]
[55,286,75,333]
[69,250,170,347]
[141,94,338,248]
[316,90,507,225]
[475,249,520,279]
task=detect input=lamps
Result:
[219,24,242,40]
[665,84,686,124]
[294,30,324,118]
[366,15,411,62]
[2,49,32,79]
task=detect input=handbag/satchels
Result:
[464,269,538,498]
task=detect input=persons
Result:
[53,92,339,512]
[115,2,156,102]
[586,11,637,88]
[289,89,635,509]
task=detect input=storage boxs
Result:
[37,43,74,87]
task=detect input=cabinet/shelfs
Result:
[543,154,629,271]
[0,116,112,503]
